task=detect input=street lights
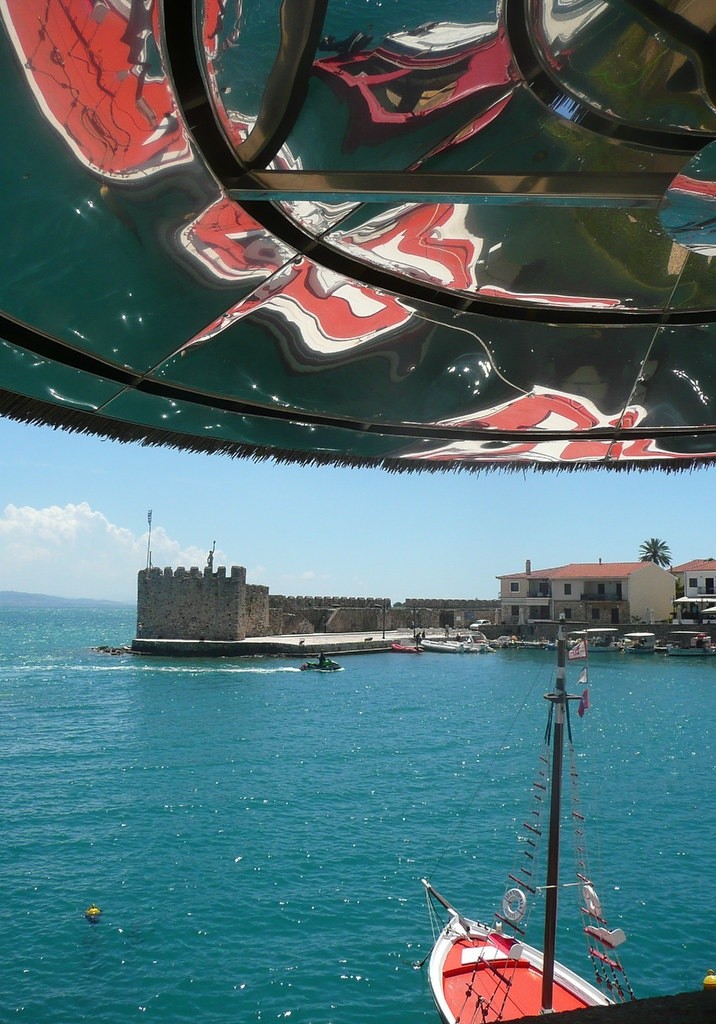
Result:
[375,605,385,639]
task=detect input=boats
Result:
[299,653,341,671]
[391,643,424,652]
[421,631,557,653]
[666,631,716,655]
[623,633,655,652]
[566,628,621,651]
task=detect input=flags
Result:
[578,689,588,718]
[576,667,589,685]
[568,640,587,660]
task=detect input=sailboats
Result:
[412,613,636,1024]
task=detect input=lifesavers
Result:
[503,888,527,922]
[583,885,601,917]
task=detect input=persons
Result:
[319,648,326,665]
[416,624,461,641]
[507,632,714,653]
[206,541,216,566]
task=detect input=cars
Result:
[469,619,491,631]
